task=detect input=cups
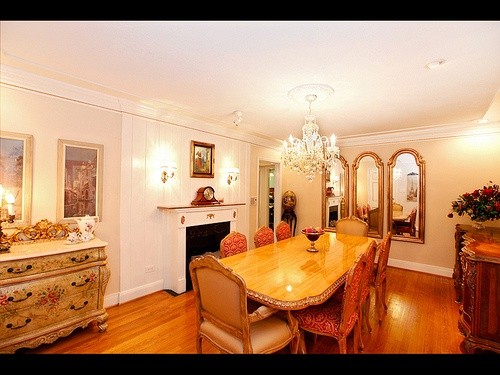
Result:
[67,232,92,243]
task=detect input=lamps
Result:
[0,193,15,252]
[233,111,244,126]
[225,168,241,185]
[279,83,341,183]
[160,164,176,183]
[190,140,215,178]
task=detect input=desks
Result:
[0,131,33,228]
[362,210,410,234]
[453,224,500,353]
[0,236,110,354]
[218,233,375,354]
[281,209,297,237]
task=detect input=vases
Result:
[472,219,486,233]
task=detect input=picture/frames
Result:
[56,139,104,224]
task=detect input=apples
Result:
[306,227,323,233]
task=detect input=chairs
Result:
[358,203,417,237]
[189,221,392,354]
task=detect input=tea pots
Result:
[74,215,99,240]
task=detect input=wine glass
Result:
[301,229,325,252]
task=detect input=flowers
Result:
[447,181,500,222]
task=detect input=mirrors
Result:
[352,151,384,239]
[321,154,349,233]
[386,148,426,245]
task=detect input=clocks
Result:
[191,186,220,205]
